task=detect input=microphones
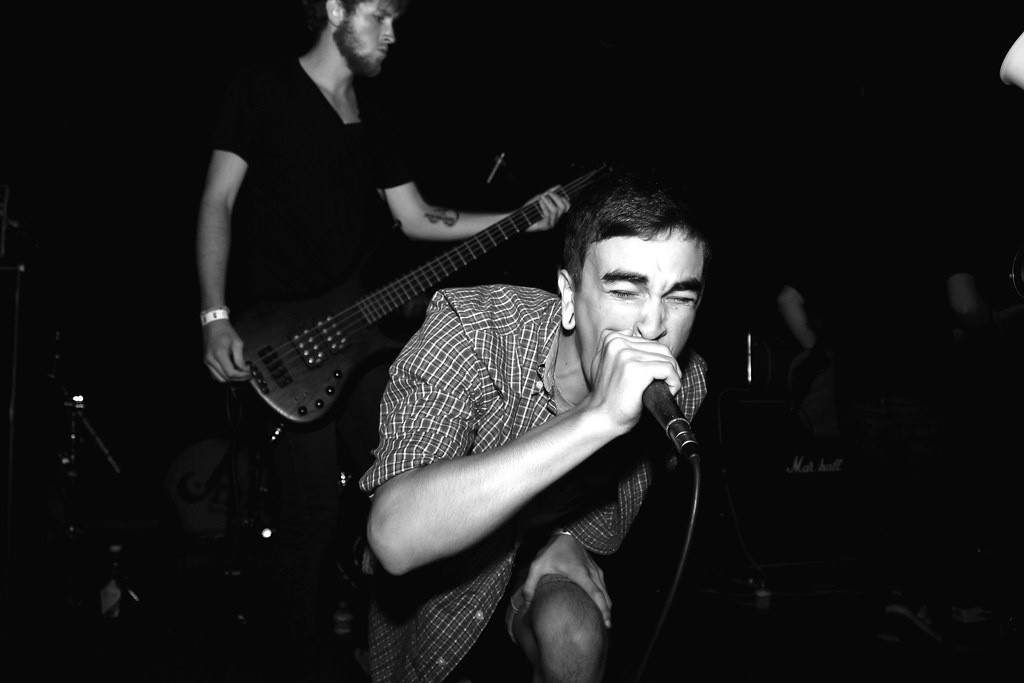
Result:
[643,380,706,470]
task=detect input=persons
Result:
[778,146,1008,646]
[195,2,570,682]
[358,173,712,683]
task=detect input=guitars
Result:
[233,158,622,426]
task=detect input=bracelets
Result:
[199,306,232,325]
[552,528,575,536]
